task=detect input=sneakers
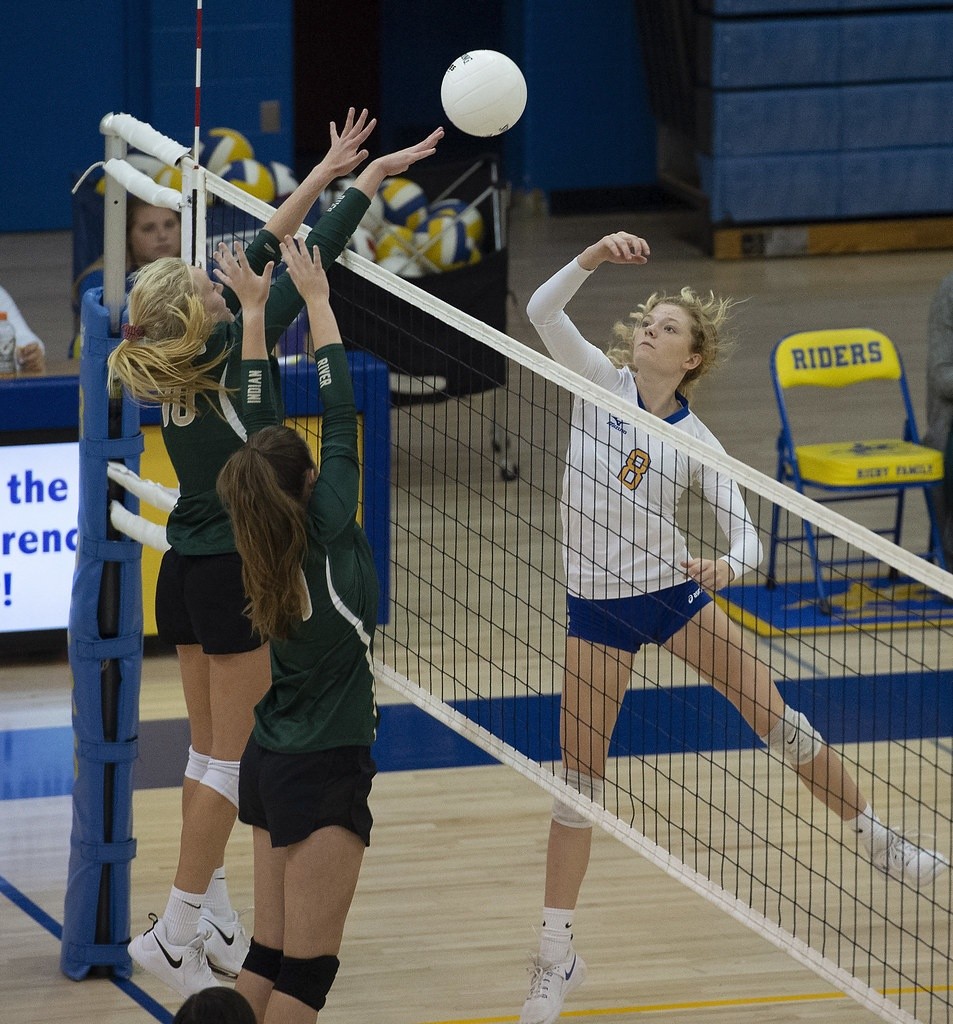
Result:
[862,826,952,887]
[521,942,587,1024]
[196,910,251,978]
[126,910,222,998]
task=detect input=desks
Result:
[0,348,391,669]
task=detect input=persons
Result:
[0,282,46,373]
[64,200,180,358]
[212,234,383,1024]
[103,101,446,982]
[171,987,257,1024]
[518,227,951,1024]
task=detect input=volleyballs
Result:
[439,49,530,139]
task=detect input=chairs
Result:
[764,325,953,617]
